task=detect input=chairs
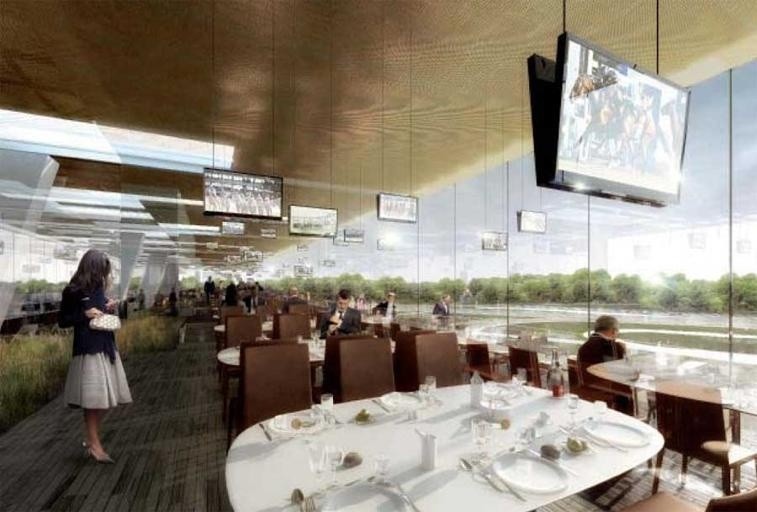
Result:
[613,369,757,509]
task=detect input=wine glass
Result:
[255,374,443,491]
[464,355,605,449]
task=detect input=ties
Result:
[338,311,343,318]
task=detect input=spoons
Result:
[290,487,306,512]
[459,458,501,492]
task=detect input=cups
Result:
[421,433,437,470]
[309,328,324,357]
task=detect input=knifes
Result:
[479,459,524,502]
[399,485,420,512]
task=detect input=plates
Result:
[583,419,650,447]
[492,452,569,493]
[321,484,407,512]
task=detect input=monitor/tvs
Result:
[525,55,554,188]
[289,205,340,237]
[377,192,418,224]
[293,244,313,276]
[516,211,546,233]
[345,230,365,243]
[202,166,284,220]
[206,223,277,264]
[557,32,702,207]
[482,231,509,251]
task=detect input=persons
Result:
[577,314,624,373]
[570,92,660,170]
[203,276,216,305]
[353,293,366,311]
[55,249,133,465]
[136,288,147,311]
[316,287,362,340]
[155,289,162,312]
[179,280,263,314]
[371,290,398,320]
[280,286,307,305]
[168,287,177,315]
[457,288,478,340]
[431,294,455,331]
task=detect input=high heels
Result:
[82,432,116,464]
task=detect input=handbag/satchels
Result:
[89,312,122,332]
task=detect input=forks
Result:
[304,496,315,512]
